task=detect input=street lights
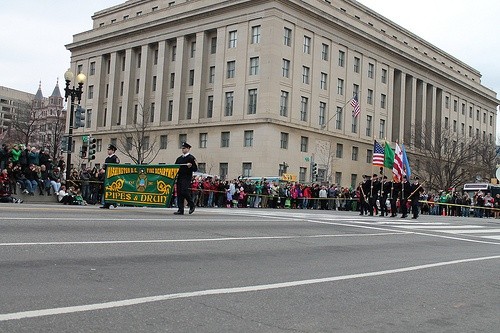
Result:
[58,71,87,190]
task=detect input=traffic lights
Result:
[310,162,317,182]
[80,136,96,162]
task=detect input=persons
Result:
[409,176,425,220]
[0,143,148,209]
[173,140,198,215]
[417,191,500,220]
[169,172,413,219]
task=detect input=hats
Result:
[362,174,420,180]
[180,141,191,150]
[107,144,117,150]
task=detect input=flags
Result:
[384,141,395,169]
[104,163,180,208]
[372,140,385,168]
[402,143,411,183]
[350,94,360,118]
[391,142,408,183]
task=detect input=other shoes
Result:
[18,199,24,204]
[99,206,109,209]
[13,199,18,203]
[173,210,184,215]
[189,202,196,214]
[63,201,88,205]
[7,190,59,196]
[359,212,420,219]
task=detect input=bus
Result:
[463,183,500,214]
[239,177,279,186]
